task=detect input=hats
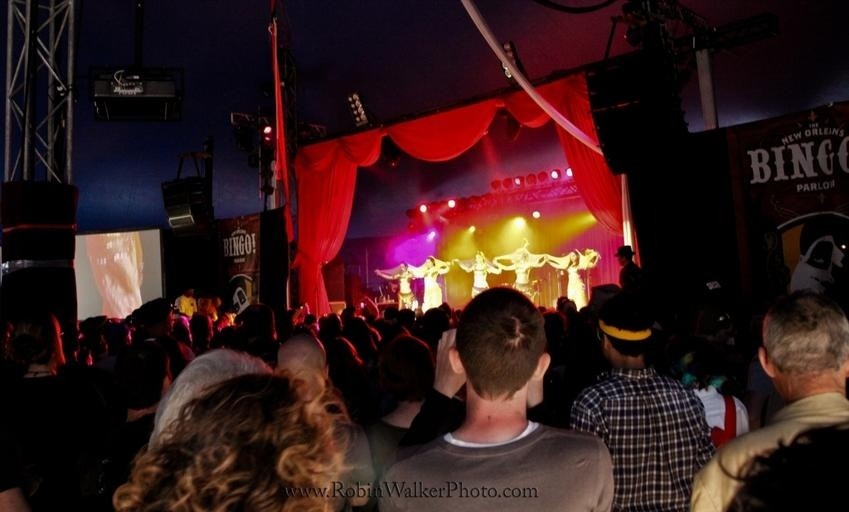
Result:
[616,246,636,258]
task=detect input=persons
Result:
[453,249,502,299]
[493,249,547,304]
[546,247,601,311]
[414,256,452,315]
[614,245,645,287]
[0,287,849,511]
[373,261,418,313]
